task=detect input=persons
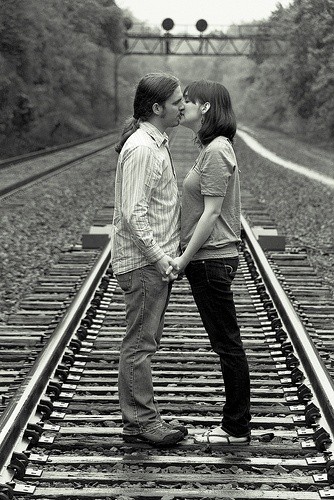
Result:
[111,72,188,445]
[162,79,253,445]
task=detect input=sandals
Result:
[194,429,251,446]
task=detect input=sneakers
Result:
[123,423,184,447]
[160,420,188,436]
